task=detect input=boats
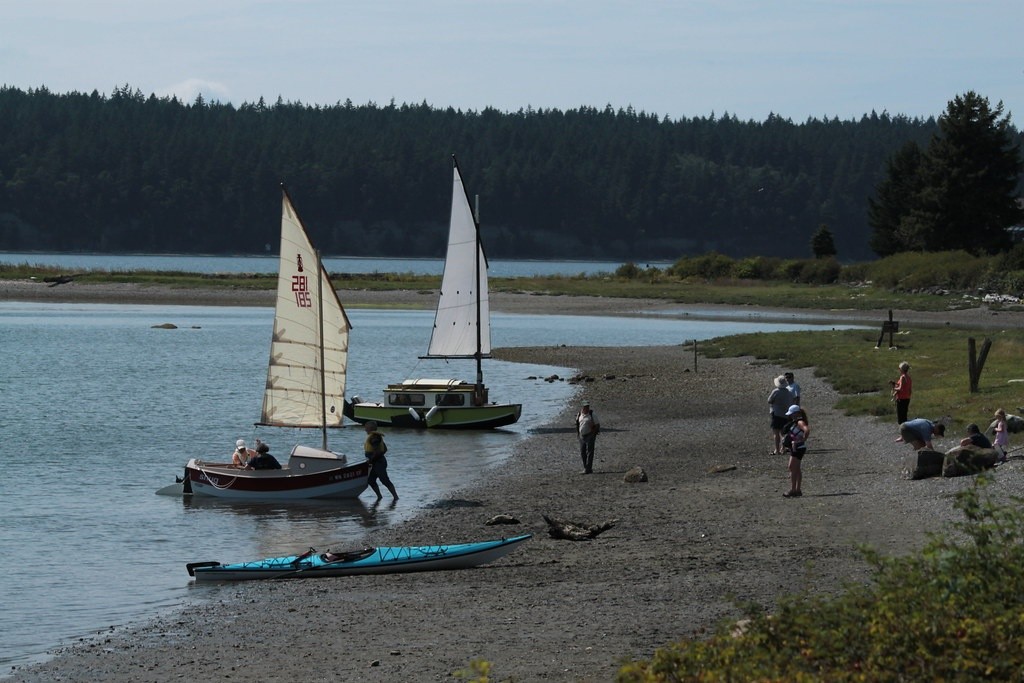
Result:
[186,532,532,577]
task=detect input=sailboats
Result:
[151,183,373,502]
[342,152,526,429]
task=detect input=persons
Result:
[894,360,911,442]
[994,406,1010,464]
[243,443,282,471]
[233,439,258,470]
[785,370,801,452]
[959,423,992,450]
[782,403,810,498]
[900,417,947,453]
[767,374,797,456]
[364,420,401,501]
[574,400,600,474]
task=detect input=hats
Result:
[785,405,800,415]
[582,400,590,406]
[938,423,945,437]
[235,439,246,449]
[899,361,910,373]
[773,375,789,388]
[994,409,1006,417]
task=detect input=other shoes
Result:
[792,491,802,496]
[782,488,794,497]
